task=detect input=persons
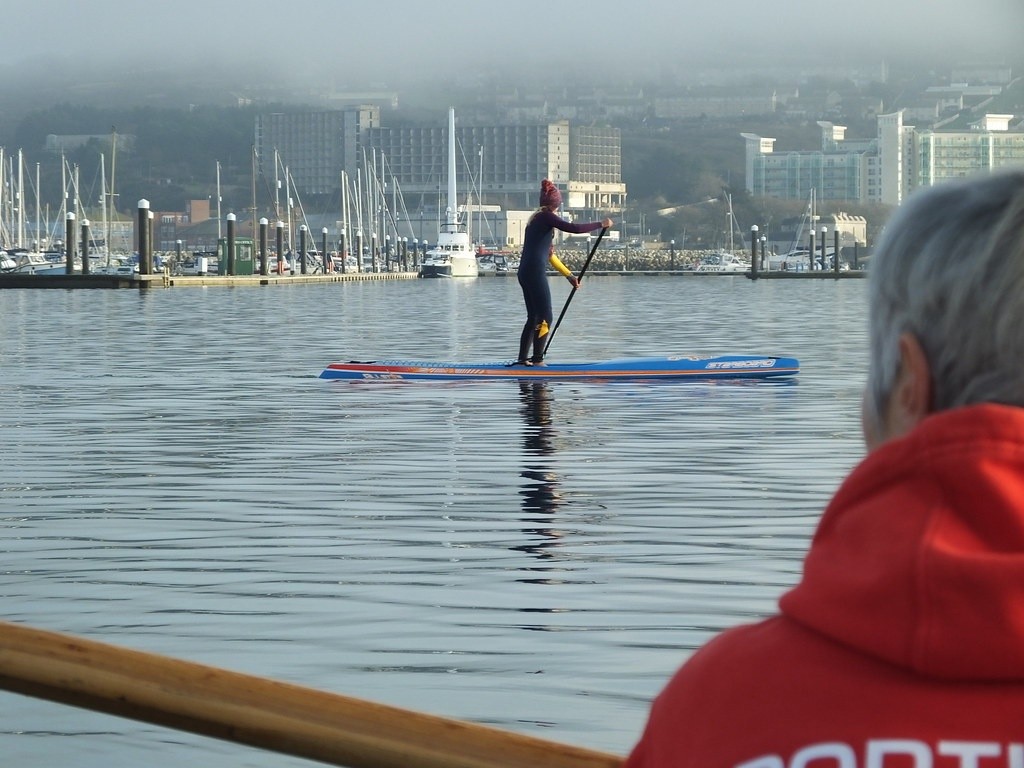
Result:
[516,180,612,367]
[627,166,1023,768]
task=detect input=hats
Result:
[539,179,561,207]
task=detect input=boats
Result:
[317,355,800,381]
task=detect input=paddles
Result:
[544,227,608,356]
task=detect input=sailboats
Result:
[0,105,510,280]
[694,189,850,275]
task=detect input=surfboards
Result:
[319,354,801,383]
